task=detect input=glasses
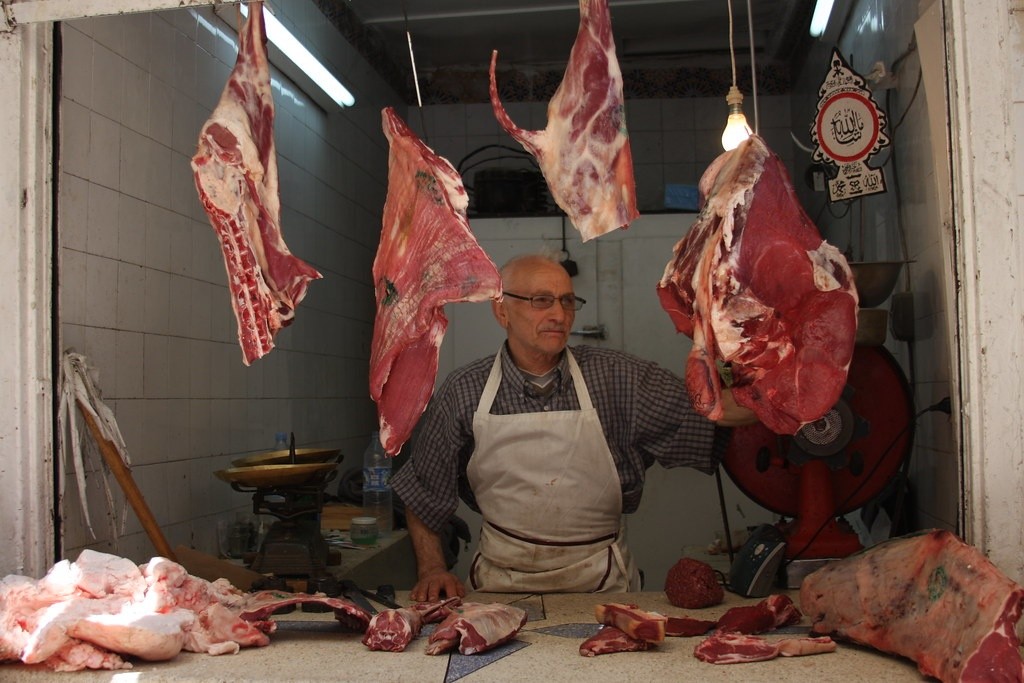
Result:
[503,290,587,311]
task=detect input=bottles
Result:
[351,517,378,545]
[273,431,289,451]
[363,431,393,538]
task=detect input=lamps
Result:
[810,0,857,42]
[214,4,355,114]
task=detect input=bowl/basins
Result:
[848,260,917,307]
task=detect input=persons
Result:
[389,256,756,602]
